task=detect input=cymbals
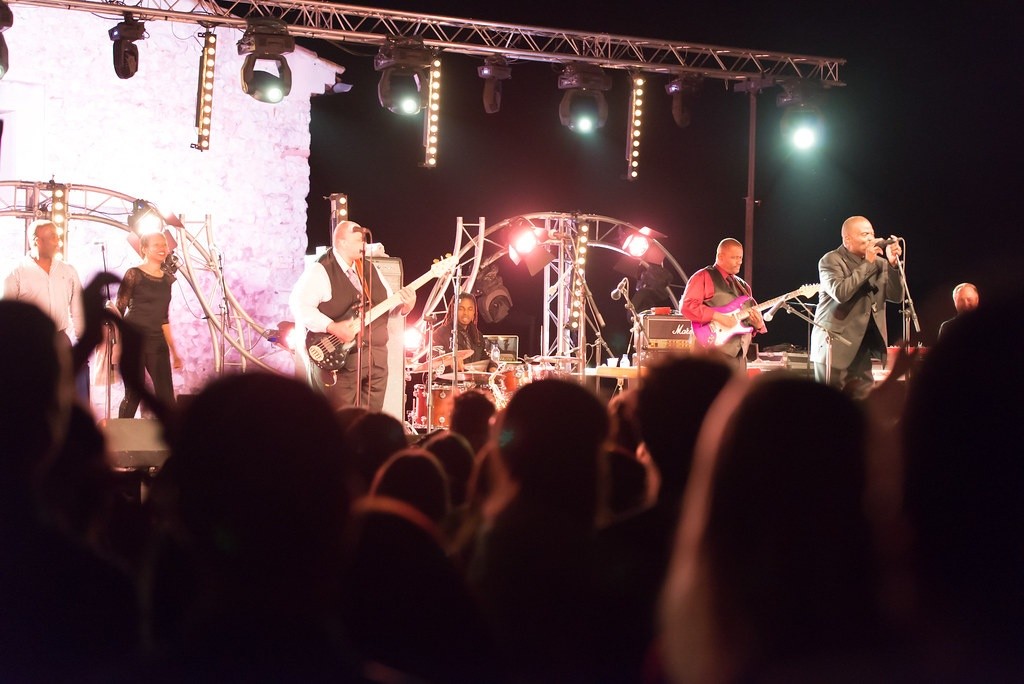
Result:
[533,355,582,363]
[414,349,475,374]
[438,369,506,382]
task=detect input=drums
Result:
[410,383,501,430]
[502,365,555,394]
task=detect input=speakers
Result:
[302,255,405,427]
[96,419,171,468]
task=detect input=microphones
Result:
[763,294,788,321]
[874,239,898,249]
[348,225,368,233]
[94,241,104,245]
[548,229,570,240]
[208,243,224,255]
[611,278,627,300]
[423,316,436,323]
[547,265,573,296]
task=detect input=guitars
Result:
[306,251,462,372]
[692,281,821,349]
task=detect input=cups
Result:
[608,358,619,368]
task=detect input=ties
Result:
[726,276,737,292]
[347,269,361,292]
[459,330,472,359]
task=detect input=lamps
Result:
[108,11,145,81]
[569,217,593,331]
[477,55,512,115]
[498,218,559,277]
[774,84,831,153]
[50,181,71,260]
[121,196,185,264]
[326,193,351,229]
[556,63,614,135]
[374,34,445,170]
[616,223,666,266]
[622,69,651,181]
[628,264,675,322]
[665,74,701,127]
[473,265,513,326]
[191,22,218,153]
[234,21,296,105]
[0,0,13,78]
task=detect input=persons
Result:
[0,216,1024,684]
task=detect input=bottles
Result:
[619,353,630,368]
[779,350,792,371]
[491,343,501,363]
